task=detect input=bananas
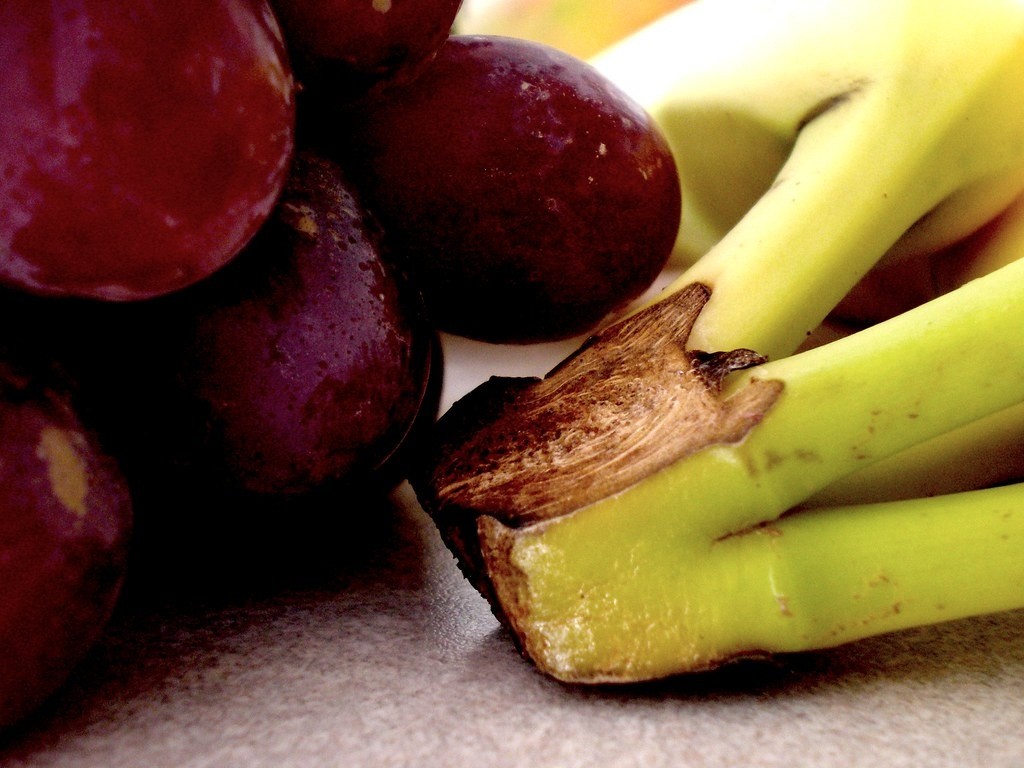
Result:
[392,0,1024,682]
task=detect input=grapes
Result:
[0,0,686,758]
